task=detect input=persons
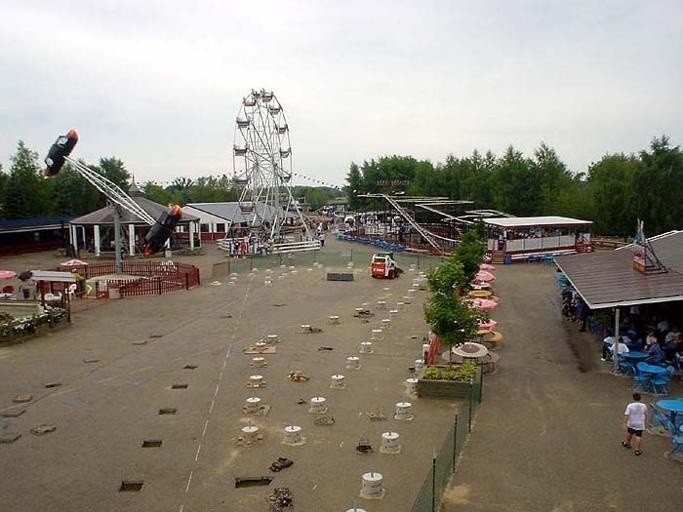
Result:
[233,239,245,258]
[72,269,85,292]
[621,393,648,455]
[319,233,324,246]
[571,286,683,372]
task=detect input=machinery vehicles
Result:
[370,254,403,279]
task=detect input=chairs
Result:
[666,422,683,455]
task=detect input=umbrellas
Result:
[473,264,496,283]
[60,259,88,268]
[475,298,498,311]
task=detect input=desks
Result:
[622,351,683,427]
